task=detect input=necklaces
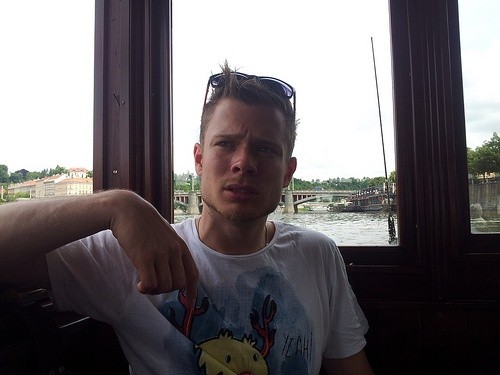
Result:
[196,216,269,248]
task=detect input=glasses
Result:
[204,71,297,114]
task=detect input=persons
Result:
[0,60,373,375]
